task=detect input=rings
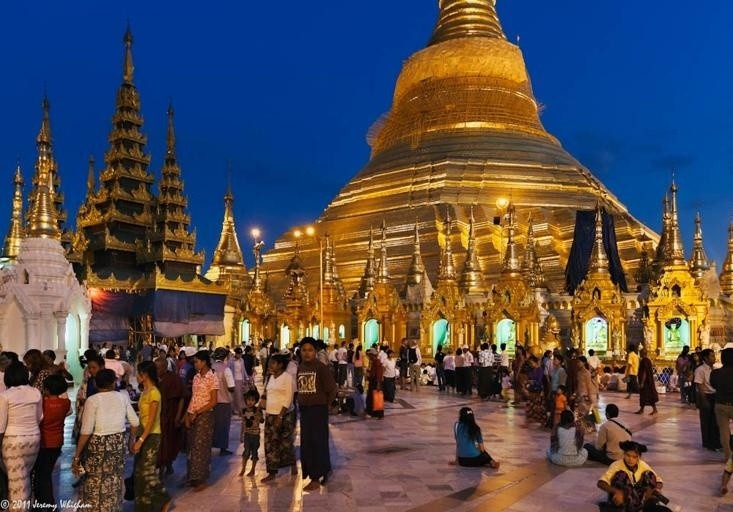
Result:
[276,424,279,426]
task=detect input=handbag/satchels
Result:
[67,462,86,487]
[396,356,401,367]
[259,374,271,410]
[593,407,602,424]
[372,389,384,411]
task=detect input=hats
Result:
[461,345,469,349]
[367,348,378,354]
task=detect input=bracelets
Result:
[139,436,144,442]
[278,415,283,419]
[73,457,80,461]
[194,410,199,417]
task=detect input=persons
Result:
[241,346,255,376]
[154,358,185,475]
[318,338,422,420]
[597,441,662,512]
[42,350,74,382]
[23,349,55,396]
[181,350,220,486]
[693,349,720,452]
[623,345,640,400]
[72,350,100,445]
[449,406,501,468]
[239,389,264,477]
[97,338,228,364]
[634,348,659,416]
[260,354,297,483]
[677,351,689,372]
[676,345,690,403]
[695,347,702,353]
[211,349,236,457]
[29,373,71,512]
[240,341,247,355]
[260,342,268,371]
[295,337,338,491]
[422,339,629,408]
[595,319,607,343]
[690,353,704,404]
[665,319,686,348]
[652,365,679,392]
[227,347,250,416]
[717,435,733,498]
[583,403,633,466]
[709,348,733,456]
[84,357,121,398]
[0,351,19,393]
[71,367,140,512]
[0,361,44,512]
[132,360,174,512]
[545,409,588,469]
